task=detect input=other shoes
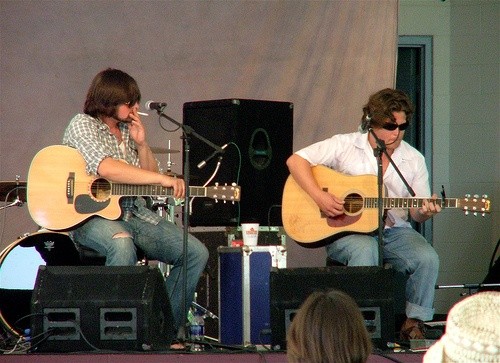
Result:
[170,343,205,351]
[400,323,424,342]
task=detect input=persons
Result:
[284,88,443,344]
[286,291,372,363]
[424,290,500,363]
[61,68,209,351]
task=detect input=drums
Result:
[0,233,77,341]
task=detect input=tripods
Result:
[159,114,258,355]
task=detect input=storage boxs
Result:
[216,245,287,352]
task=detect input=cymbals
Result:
[161,169,185,179]
[0,179,27,204]
[149,145,183,155]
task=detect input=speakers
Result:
[30,266,176,351]
[269,266,407,353]
[182,99,294,227]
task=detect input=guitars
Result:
[280,163,493,250]
[25,145,241,232]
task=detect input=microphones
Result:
[145,100,168,111]
[357,114,371,135]
[198,142,228,170]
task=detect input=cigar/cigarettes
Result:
[137,111,148,117]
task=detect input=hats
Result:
[423,291,500,363]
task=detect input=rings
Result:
[428,210,431,211]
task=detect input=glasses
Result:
[383,121,409,130]
[122,94,141,108]
